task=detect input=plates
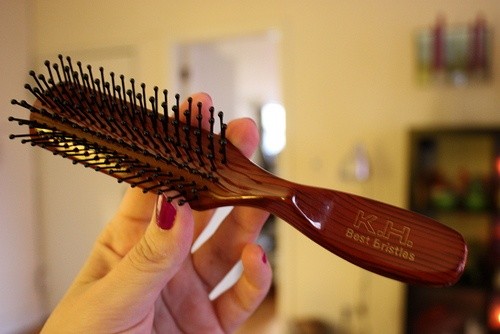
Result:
[404,126,500,334]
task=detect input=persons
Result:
[37,92,274,333]
